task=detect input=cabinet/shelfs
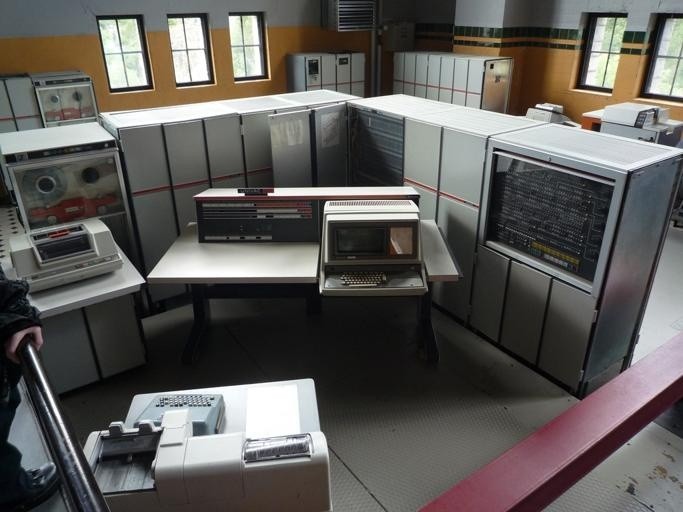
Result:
[392,51,513,114]
[287,53,366,99]
[0,73,44,132]
[0,241,148,400]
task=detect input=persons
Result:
[0,264,62,512]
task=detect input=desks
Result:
[145,220,463,370]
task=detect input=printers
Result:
[83,379,334,512]
[7,217,124,293]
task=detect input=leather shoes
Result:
[8,462,60,511]
